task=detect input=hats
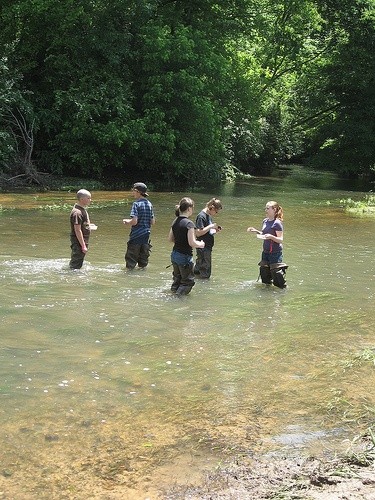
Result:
[133,182,150,196]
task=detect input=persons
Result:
[122,183,157,269]
[193,197,222,278]
[169,197,206,295]
[70,188,97,268]
[247,201,288,289]
